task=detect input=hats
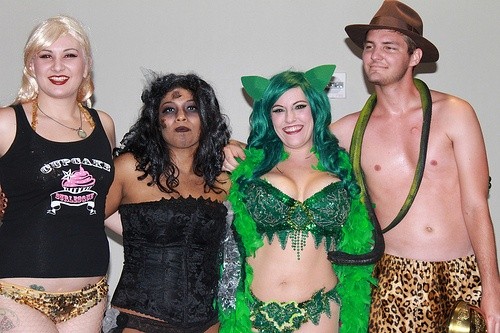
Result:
[345,0,439,64]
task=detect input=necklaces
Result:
[35,99,87,139]
[276,153,315,175]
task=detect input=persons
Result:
[0,69,230,333]
[213,65,377,333]
[221,0,500,333]
[0,13,116,333]
